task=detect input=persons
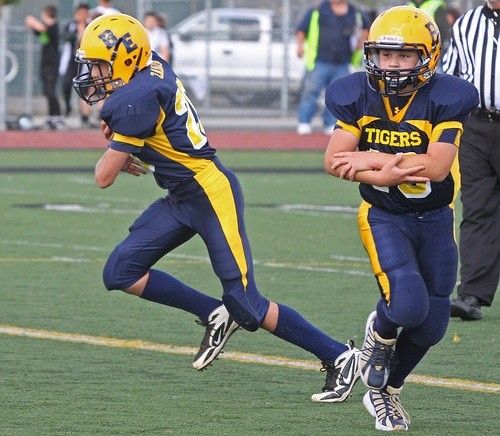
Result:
[74,14,363,403]
[26,0,173,126]
[443,0,500,320]
[325,5,478,431]
[295,0,462,134]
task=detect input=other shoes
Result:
[297,122,313,135]
[450,296,481,321]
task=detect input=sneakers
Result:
[311,339,361,403]
[358,310,398,390]
[362,384,410,431]
[192,303,241,371]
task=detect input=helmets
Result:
[362,6,441,97]
[73,13,151,105]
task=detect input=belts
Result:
[471,108,500,120]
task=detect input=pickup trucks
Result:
[170,7,305,97]
